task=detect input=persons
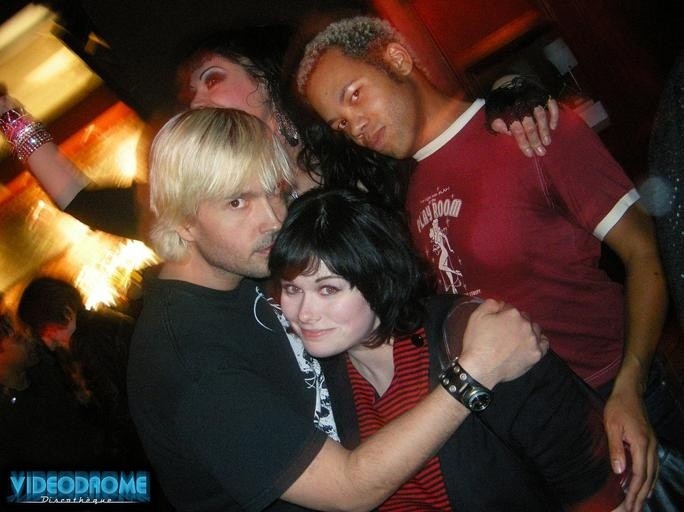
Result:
[296,14,668,512]
[16,275,136,451]
[123,106,551,512]
[268,184,684,511]
[0,29,559,287]
[0,313,93,474]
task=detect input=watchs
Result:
[437,357,493,414]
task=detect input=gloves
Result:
[484,71,553,135]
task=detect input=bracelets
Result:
[0,104,54,164]
